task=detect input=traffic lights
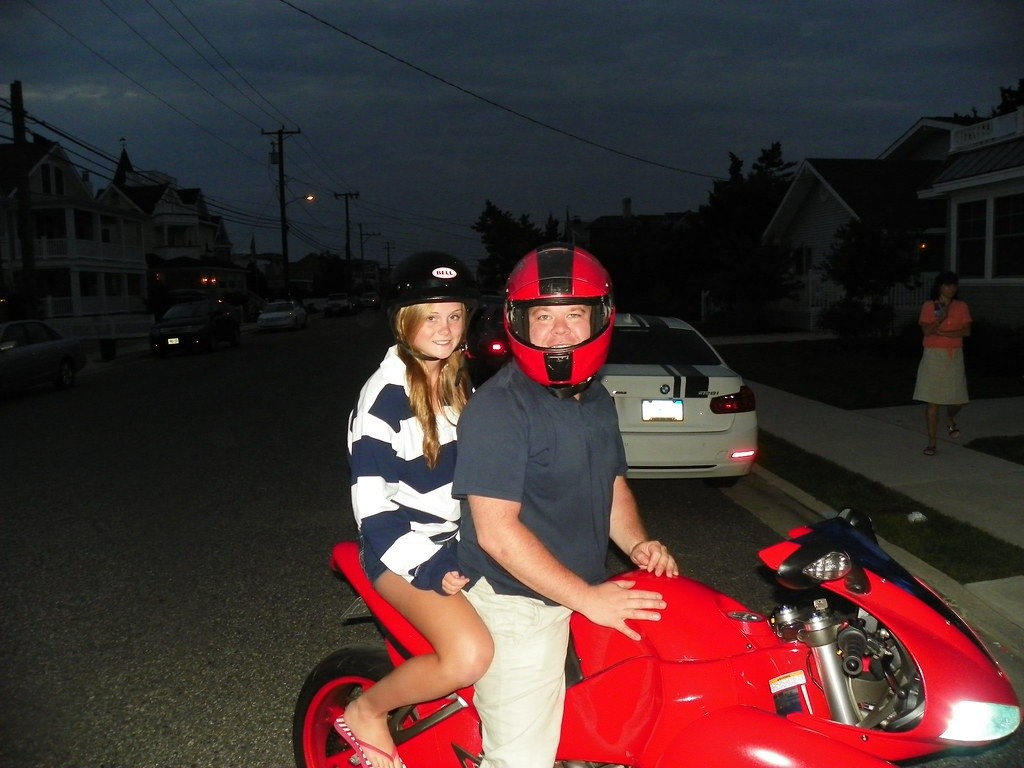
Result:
[200,271,218,290]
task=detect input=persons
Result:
[912,270,973,455]
[331,252,495,768]
[451,245,678,768]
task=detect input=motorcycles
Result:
[290,506,1024,768]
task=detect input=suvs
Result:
[147,297,242,358]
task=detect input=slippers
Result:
[947,422,959,438]
[334,713,407,768]
[923,445,936,455]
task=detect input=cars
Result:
[256,300,307,332]
[598,312,760,488]
[0,318,86,393]
[460,302,514,391]
[358,292,379,308]
[323,293,354,318]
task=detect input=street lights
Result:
[279,194,314,297]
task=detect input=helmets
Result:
[502,242,615,387]
[383,249,480,320]
